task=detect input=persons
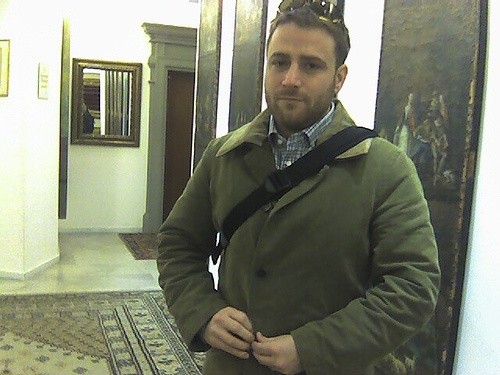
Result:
[156,1,444,374]
[83,102,95,134]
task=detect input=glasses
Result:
[276,0,344,25]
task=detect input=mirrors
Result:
[70,57,143,147]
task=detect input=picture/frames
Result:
[0,39,10,97]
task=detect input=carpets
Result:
[0,290,206,375]
[118,232,159,260]
